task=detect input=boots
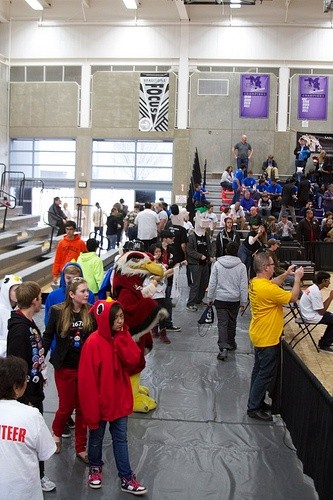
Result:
[152,325,159,337]
[158,328,171,344]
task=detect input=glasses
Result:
[25,375,30,383]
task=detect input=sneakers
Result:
[41,476,56,492]
[120,473,149,495]
[88,466,102,488]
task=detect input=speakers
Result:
[16,187,32,214]
[134,189,155,203]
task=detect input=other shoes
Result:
[166,325,182,332]
[76,452,89,463]
[317,343,333,353]
[198,301,208,308]
[217,349,227,360]
[61,424,72,438]
[185,305,198,312]
[52,431,63,453]
[68,415,75,428]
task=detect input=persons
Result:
[231,134,254,171]
[299,265,333,351]
[44,262,97,437]
[247,251,304,421]
[42,276,98,464]
[78,296,149,496]
[48,150,333,311]
[294,138,311,173]
[0,354,57,500]
[0,272,56,491]
[206,242,249,361]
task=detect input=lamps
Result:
[121,0,139,10]
[78,181,87,188]
[24,0,44,11]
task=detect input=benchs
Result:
[0,257,55,292]
[100,249,120,268]
[48,231,82,248]
[0,233,18,248]
[4,214,41,229]
[0,245,42,271]
[25,224,58,238]
[0,205,23,219]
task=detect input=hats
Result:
[268,238,280,244]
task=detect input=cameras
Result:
[294,266,299,271]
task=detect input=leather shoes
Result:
[259,401,270,412]
[247,409,273,421]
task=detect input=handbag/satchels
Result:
[198,304,214,325]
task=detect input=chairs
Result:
[268,264,325,354]
[222,179,333,228]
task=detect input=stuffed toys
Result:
[112,251,164,413]
